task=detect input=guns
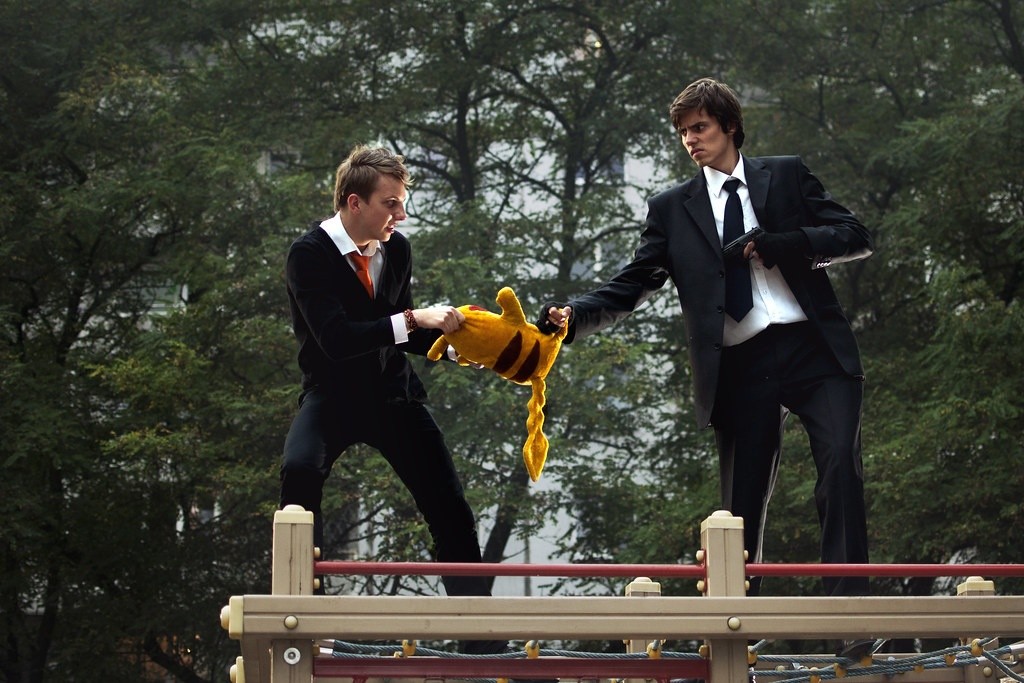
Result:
[723,225,776,269]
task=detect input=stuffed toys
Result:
[425,287,569,482]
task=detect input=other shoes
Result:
[834,640,876,655]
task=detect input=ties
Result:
[349,252,375,301]
[722,176,754,323]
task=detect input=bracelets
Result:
[403,308,418,333]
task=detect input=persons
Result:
[279,146,516,655]
[536,77,875,658]
